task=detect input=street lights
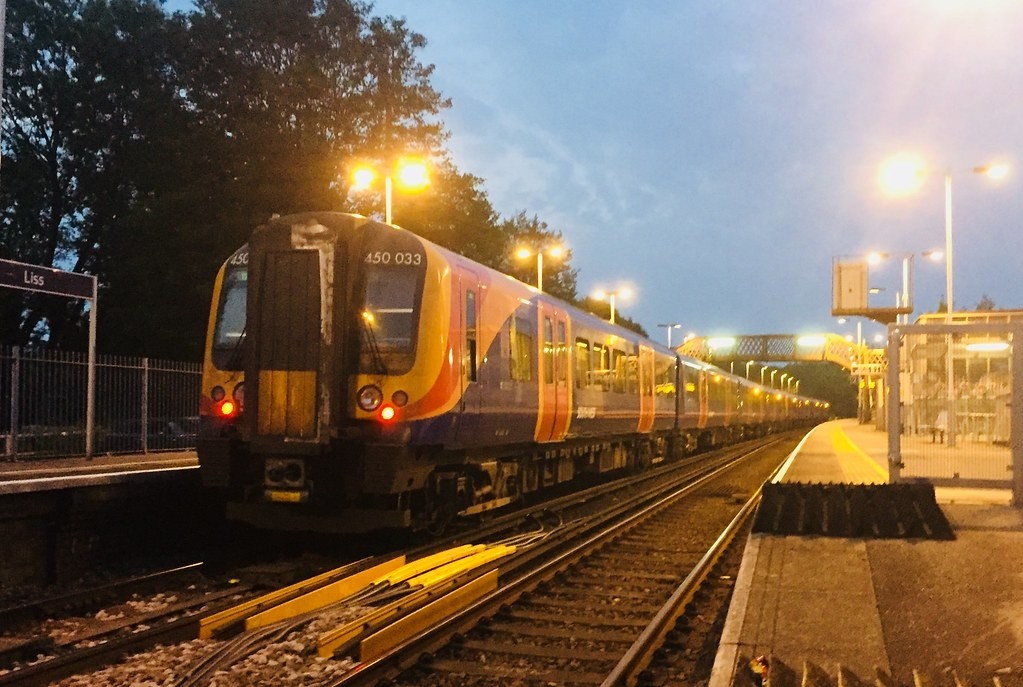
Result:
[888,159,1009,449]
[868,249,944,325]
[796,380,800,394]
[595,284,633,325]
[845,335,883,347]
[838,317,876,424]
[869,288,900,325]
[746,360,754,381]
[351,158,428,224]
[788,376,793,393]
[760,366,768,385]
[657,323,681,349]
[781,374,787,391]
[517,246,563,292]
[706,337,734,365]
[770,369,777,388]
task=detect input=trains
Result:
[193,211,828,548]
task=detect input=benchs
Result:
[923,411,949,443]
